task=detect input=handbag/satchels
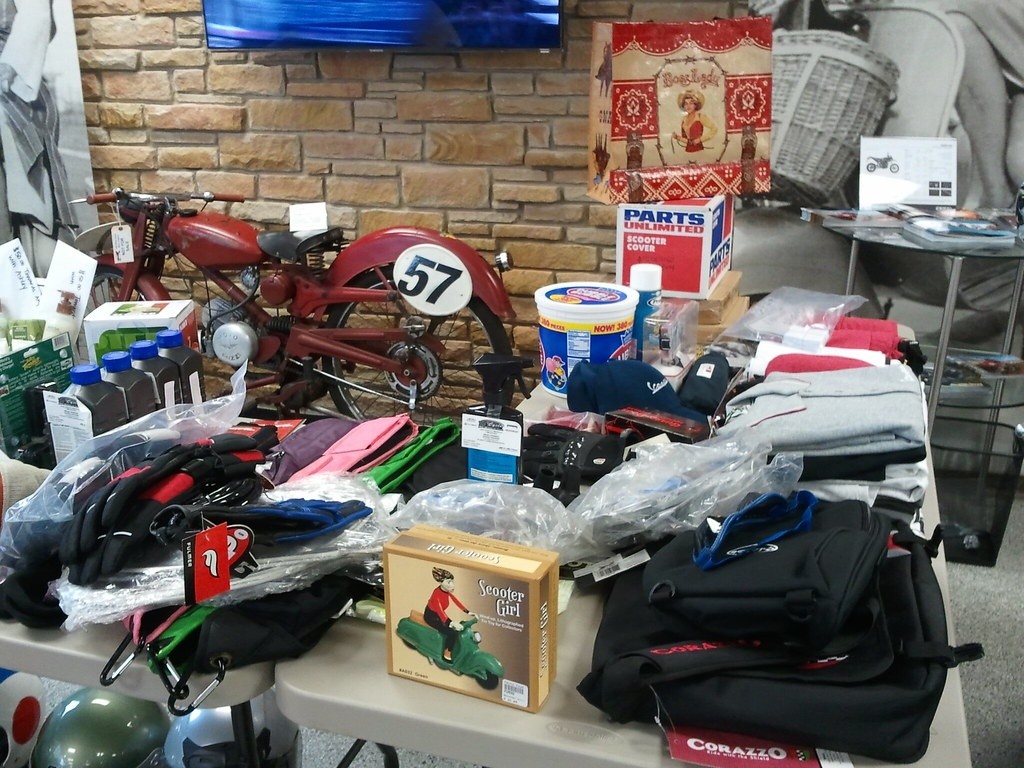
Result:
[127,568,368,716]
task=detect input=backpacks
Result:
[577,497,984,763]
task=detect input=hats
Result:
[566,358,710,424]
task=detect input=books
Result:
[888,205,1017,243]
[800,207,904,228]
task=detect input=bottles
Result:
[129,339,183,410]
[62,362,127,436]
[101,350,156,424]
[155,329,206,406]
[629,264,663,361]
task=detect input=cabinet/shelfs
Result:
[825,216,1024,532]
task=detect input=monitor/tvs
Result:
[202,0,565,53]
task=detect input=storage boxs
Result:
[649,271,742,323]
[0,332,75,470]
[378,525,558,712]
[641,300,699,388]
[84,297,195,366]
[860,134,957,206]
[654,299,750,345]
[618,192,734,297]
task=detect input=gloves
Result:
[522,423,622,500]
[145,495,373,548]
[61,430,262,583]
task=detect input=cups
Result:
[535,280,640,398]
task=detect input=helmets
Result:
[163,690,299,767]
[29,686,172,767]
[0,668,43,768]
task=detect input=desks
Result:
[0,381,970,768]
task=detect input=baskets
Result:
[928,417,1024,565]
[758,27,899,206]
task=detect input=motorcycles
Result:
[86,187,531,421]
